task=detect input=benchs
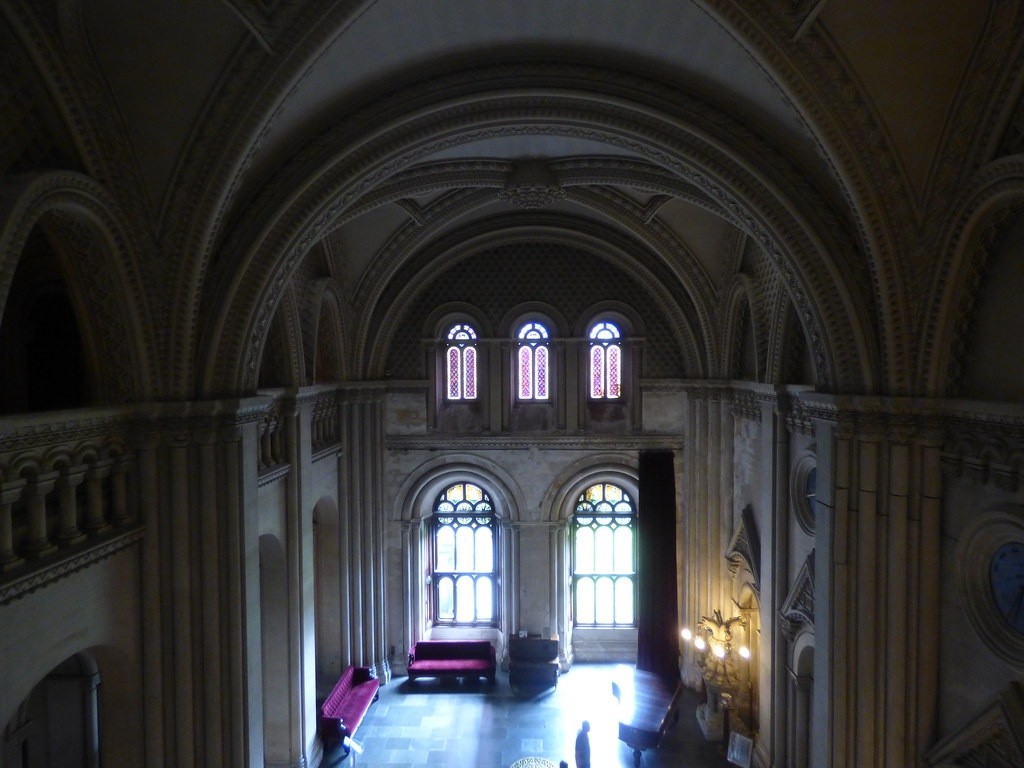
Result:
[508,637,559,687]
[407,642,496,688]
[316,665,380,756]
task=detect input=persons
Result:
[575,720,590,768]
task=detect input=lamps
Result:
[680,610,754,743]
